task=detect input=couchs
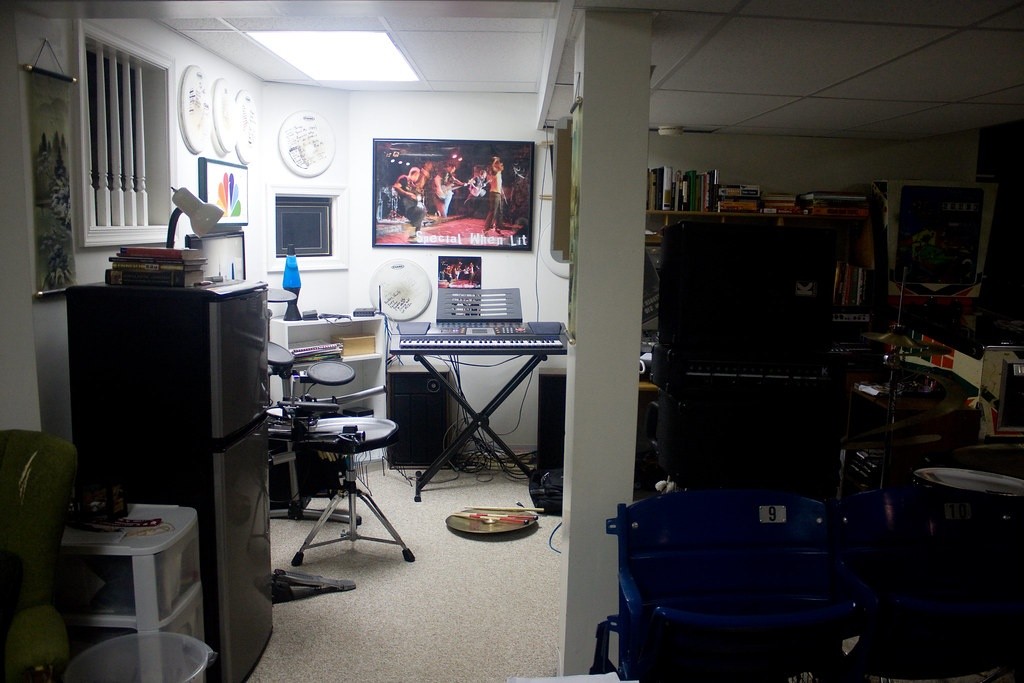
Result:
[0,428,79,683]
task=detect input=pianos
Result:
[396,321,569,350]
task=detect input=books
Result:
[106,243,207,286]
[646,165,869,218]
[833,258,867,307]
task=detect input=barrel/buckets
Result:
[64,632,214,683]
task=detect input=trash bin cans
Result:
[62,631,211,683]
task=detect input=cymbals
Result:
[445,508,539,534]
[859,329,930,351]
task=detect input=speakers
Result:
[536,367,567,470]
[387,363,460,468]
[659,218,843,500]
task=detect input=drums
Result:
[904,466,1024,526]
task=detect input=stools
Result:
[285,416,417,567]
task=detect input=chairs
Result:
[588,480,1023,683]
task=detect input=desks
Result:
[390,335,568,501]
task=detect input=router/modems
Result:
[353,285,381,317]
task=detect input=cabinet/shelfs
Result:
[269,314,390,467]
[639,209,870,391]
[59,499,206,682]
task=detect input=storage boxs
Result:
[331,332,375,355]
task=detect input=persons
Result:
[394,157,508,237]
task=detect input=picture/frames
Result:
[372,141,537,251]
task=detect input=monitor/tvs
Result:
[185,230,246,283]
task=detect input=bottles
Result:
[282,244,302,321]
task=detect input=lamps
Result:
[165,187,224,247]
